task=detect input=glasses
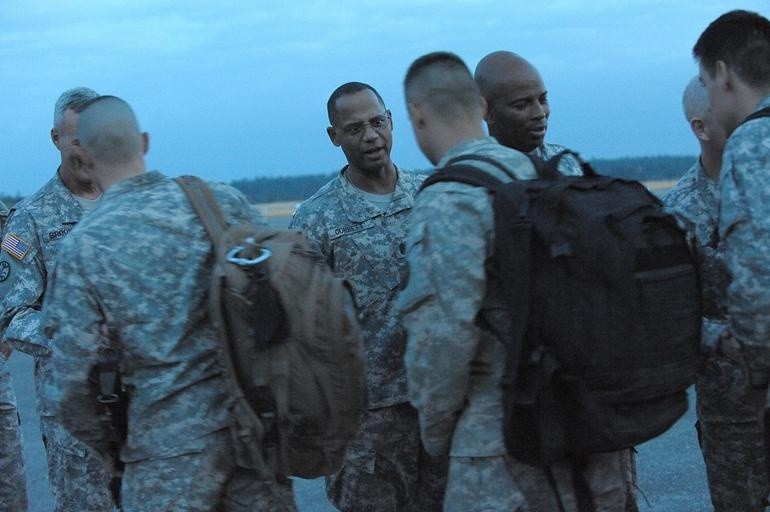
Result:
[339,114,389,135]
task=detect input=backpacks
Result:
[417,149,702,466]
[173,174,369,481]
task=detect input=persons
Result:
[0,201,30,511]
[395,52,628,512]
[0,87,129,511]
[661,76,770,512]
[475,50,639,511]
[33,94,298,512]
[693,9,770,348]
[286,81,468,511]
